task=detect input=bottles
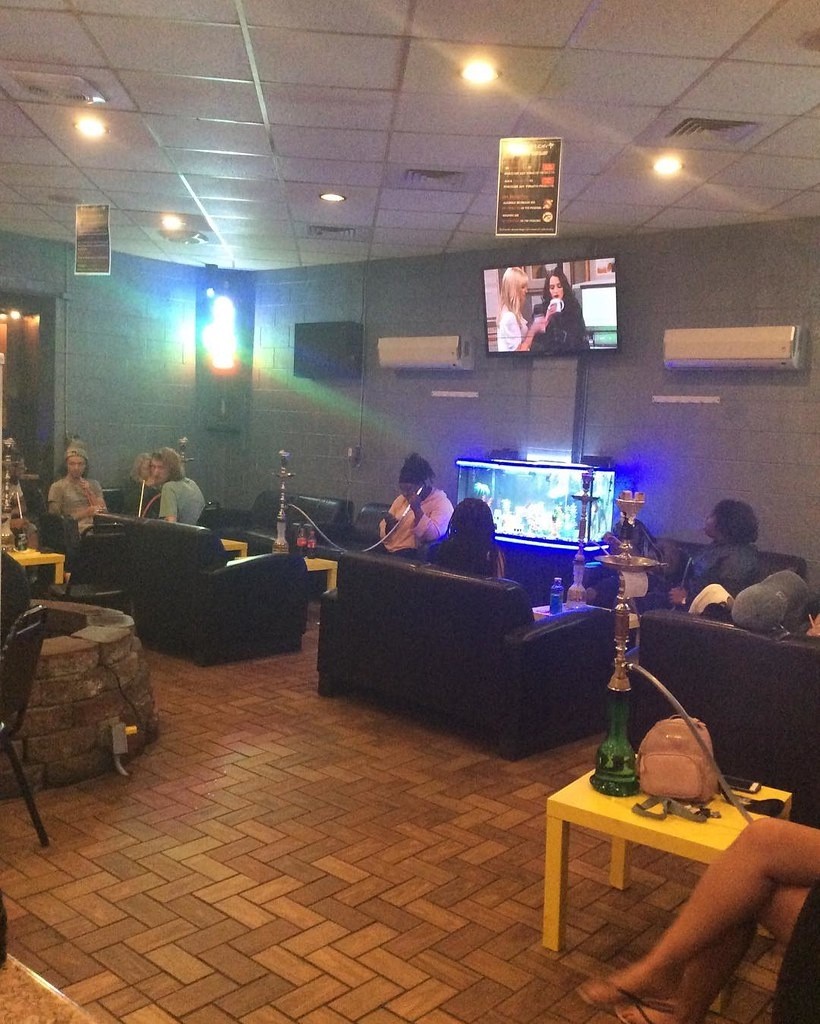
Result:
[307,530,316,559]
[17,522,39,551]
[296,528,307,559]
[549,577,564,614]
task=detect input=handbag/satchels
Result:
[635,714,721,823]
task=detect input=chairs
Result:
[0,604,51,850]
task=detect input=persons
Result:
[578,817,820,1024]
[586,519,666,612]
[733,570,820,638]
[46,446,107,534]
[123,452,161,519]
[375,453,454,559]
[426,497,506,578]
[149,447,206,526]
[669,499,758,612]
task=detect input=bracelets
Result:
[682,598,686,605]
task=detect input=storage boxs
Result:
[453,459,616,550]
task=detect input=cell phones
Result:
[723,775,761,792]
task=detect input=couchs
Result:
[40,489,820,830]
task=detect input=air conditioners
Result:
[377,336,478,374]
[662,324,808,373]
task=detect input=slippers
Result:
[612,1000,674,1024]
[578,976,643,1009]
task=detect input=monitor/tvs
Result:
[482,257,619,357]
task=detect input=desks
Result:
[532,603,611,623]
[220,539,248,559]
[542,754,793,1014]
[303,556,339,592]
[7,549,67,585]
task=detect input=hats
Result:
[66,447,88,463]
[731,570,809,635]
